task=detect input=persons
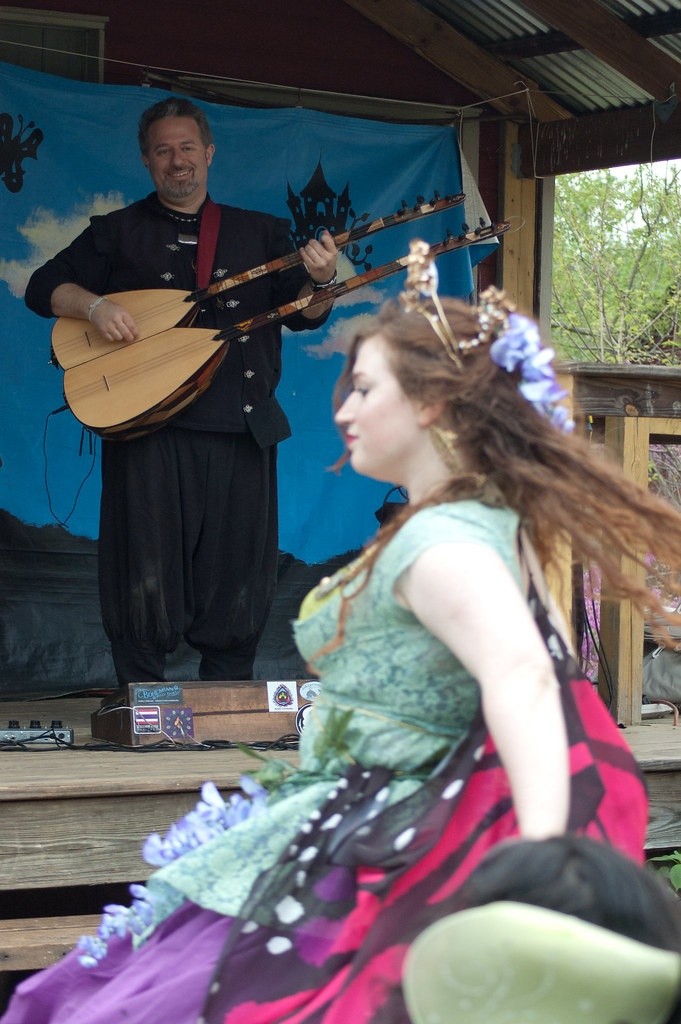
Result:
[0,241,681,1024]
[25,99,340,699]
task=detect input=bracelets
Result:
[311,269,338,293]
[87,296,107,322]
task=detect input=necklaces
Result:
[164,210,199,224]
